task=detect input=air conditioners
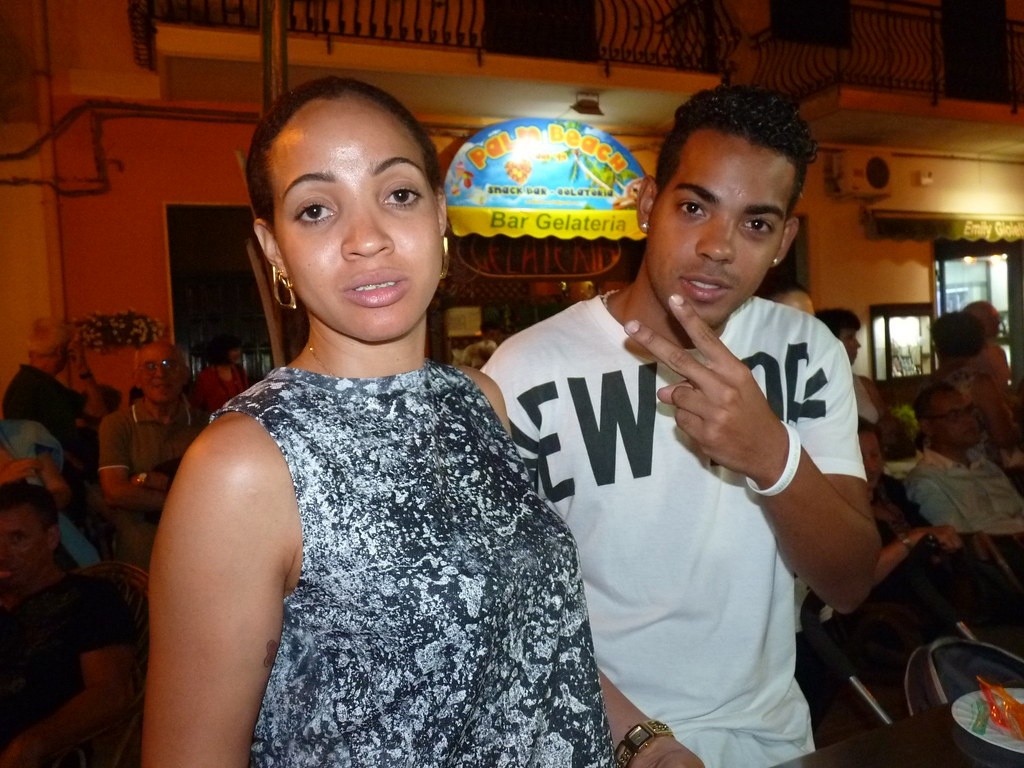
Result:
[828,147,895,202]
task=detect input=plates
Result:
[951,688,1024,753]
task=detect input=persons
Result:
[479,82,883,768]
[0,278,1024,768]
[141,77,707,768]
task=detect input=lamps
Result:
[571,92,604,116]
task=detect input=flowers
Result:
[70,310,165,350]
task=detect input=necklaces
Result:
[306,343,337,379]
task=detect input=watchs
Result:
[136,471,147,488]
[615,720,673,768]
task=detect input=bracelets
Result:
[745,420,802,497]
[77,369,92,377]
[897,533,913,549]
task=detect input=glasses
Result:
[135,358,188,373]
[924,404,979,423]
